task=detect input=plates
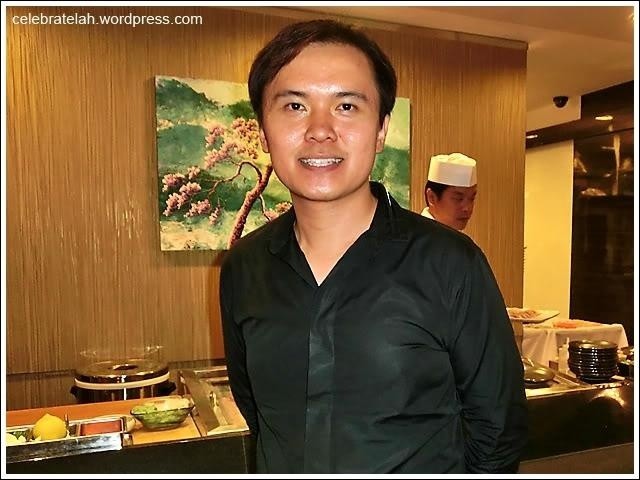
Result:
[567,337,620,382]
[506,307,561,325]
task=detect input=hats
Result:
[427,152,477,188]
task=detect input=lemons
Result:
[32,413,66,441]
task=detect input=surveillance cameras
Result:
[553,97,568,107]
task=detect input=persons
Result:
[418,151,477,231]
[220,19,526,473]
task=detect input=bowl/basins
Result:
[129,397,195,431]
